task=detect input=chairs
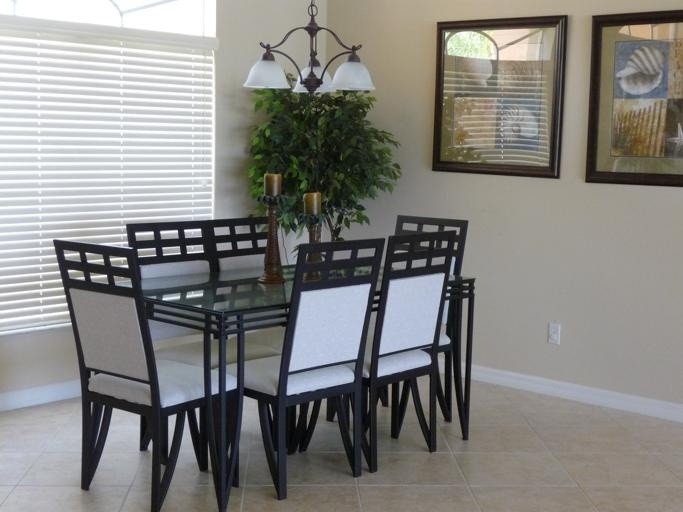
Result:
[213,236,388,501]
[54,238,244,511]
[361,229,457,475]
[122,215,325,455]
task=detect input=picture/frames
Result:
[432,14,569,181]
[584,8,683,186]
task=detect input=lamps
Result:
[242,0,378,93]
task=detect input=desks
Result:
[116,265,476,487]
